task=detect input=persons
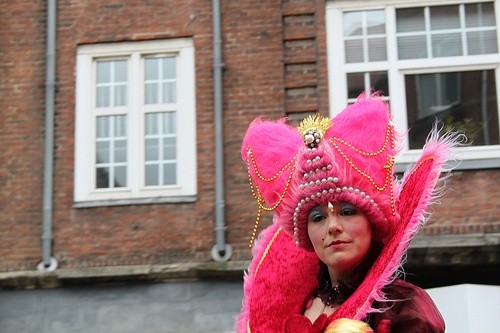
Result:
[234,93,446,333]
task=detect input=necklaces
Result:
[316,278,363,308]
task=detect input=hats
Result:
[242,96,398,252]
